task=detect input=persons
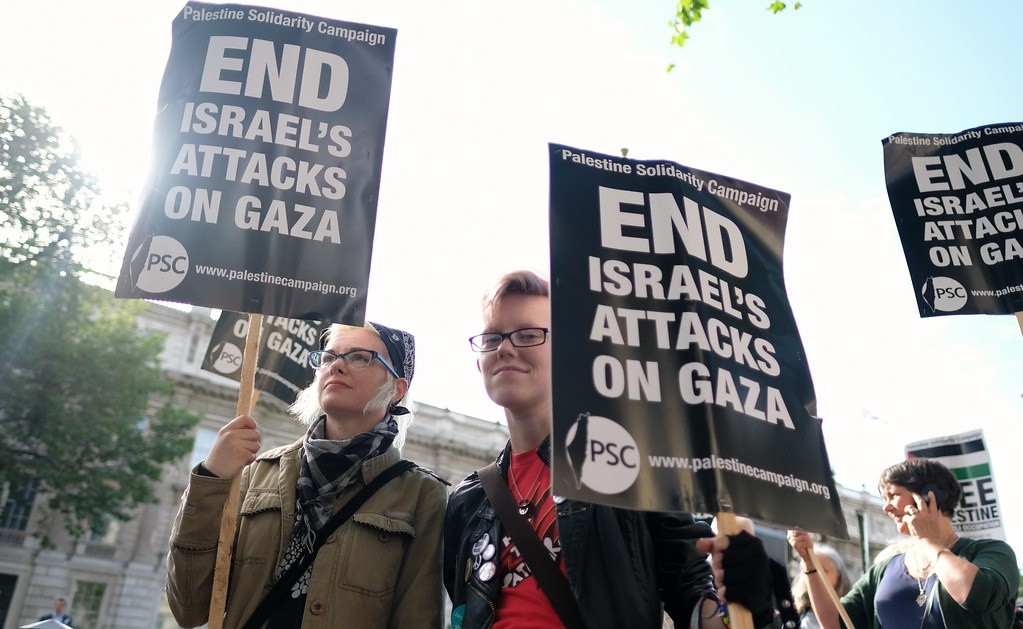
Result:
[786,460,1018,629]
[441,269,801,629]
[38,598,72,627]
[1012,607,1023,629]
[166,319,447,629]
[705,514,852,629]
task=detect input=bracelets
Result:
[805,569,817,574]
[936,549,950,557]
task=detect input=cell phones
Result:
[912,483,945,509]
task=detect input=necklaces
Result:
[510,450,545,516]
[912,530,960,606]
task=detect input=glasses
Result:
[469,328,550,352]
[307,350,399,379]
